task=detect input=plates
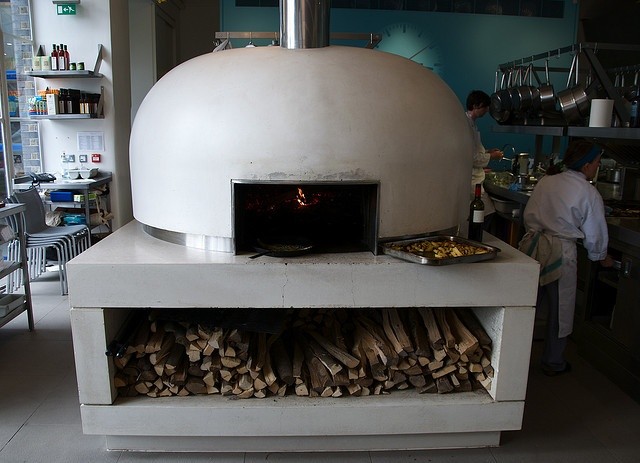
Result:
[381,234,501,267]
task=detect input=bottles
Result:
[69,63,76,71]
[513,155,520,176]
[468,183,484,242]
[58,88,66,113]
[83,94,90,114]
[518,153,529,174]
[58,44,69,70]
[79,93,84,114]
[630,87,640,128]
[78,62,84,70]
[93,93,103,116]
[50,43,59,70]
[66,88,75,113]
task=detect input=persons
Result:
[518,137,615,375]
[463,90,504,236]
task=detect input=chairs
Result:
[0,186,94,295]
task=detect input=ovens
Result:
[584,256,631,333]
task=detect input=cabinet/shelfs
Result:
[0,203,34,333]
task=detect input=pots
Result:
[488,62,539,125]
[539,58,557,112]
[248,243,314,260]
[557,52,592,125]
[604,165,622,183]
